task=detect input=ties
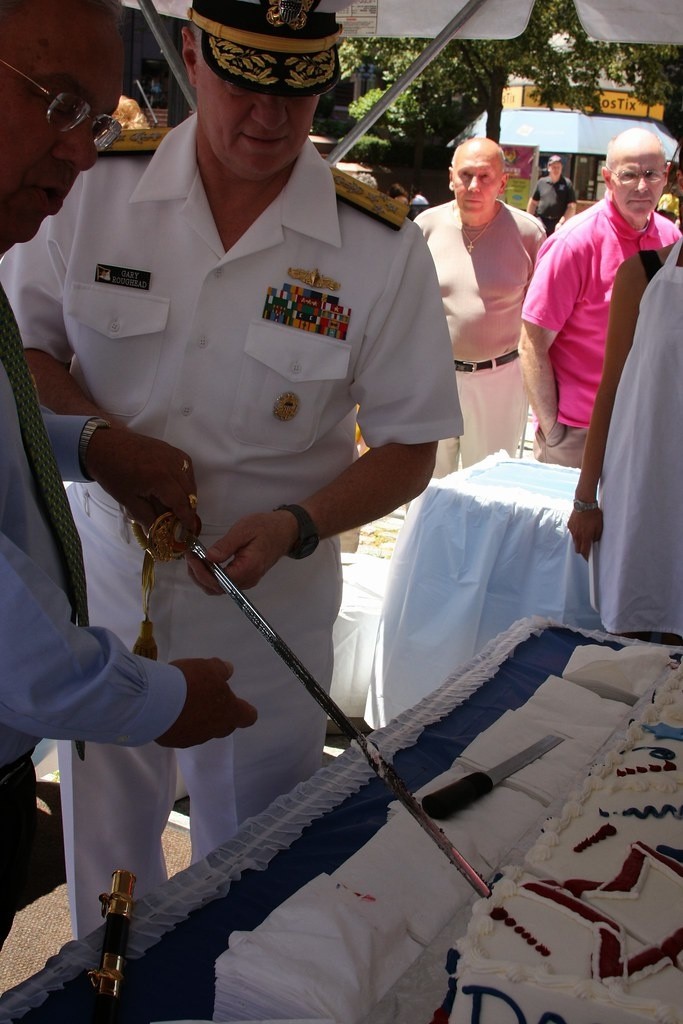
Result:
[1,284,89,762]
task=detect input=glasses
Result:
[605,166,666,183]
[0,58,122,153]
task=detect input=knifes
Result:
[422,734,565,820]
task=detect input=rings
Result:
[182,460,189,472]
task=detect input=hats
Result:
[188,0,343,97]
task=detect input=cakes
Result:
[427,655,683,1024]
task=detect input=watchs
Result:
[78,417,112,469]
[273,504,319,560]
[573,500,598,513]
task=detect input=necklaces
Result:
[457,199,498,253]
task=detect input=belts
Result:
[455,349,519,374]
[0,748,34,788]
[538,214,560,222]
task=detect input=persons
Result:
[406,137,547,515]
[0,0,258,951]
[567,140,683,645]
[526,155,576,238]
[384,182,430,205]
[656,186,680,229]
[0,0,464,941]
[518,128,682,468]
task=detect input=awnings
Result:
[445,106,681,163]
[122,1,682,168]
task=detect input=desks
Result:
[364,449,600,728]
[0,613,683,1024]
